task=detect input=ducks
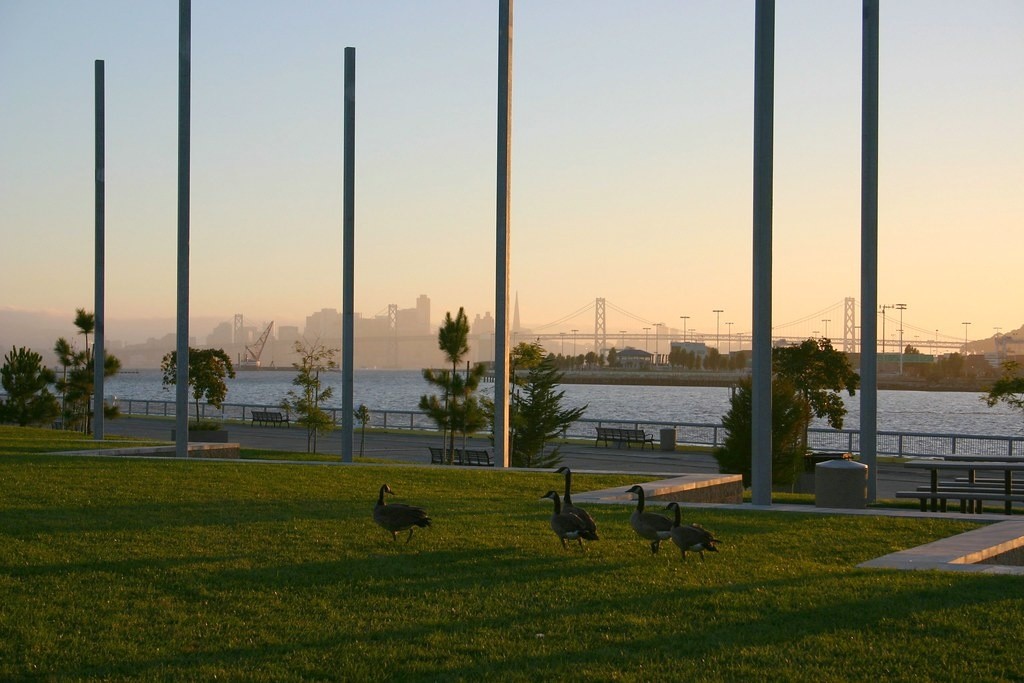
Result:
[663,502,723,563]
[541,490,589,552]
[553,466,600,547]
[624,485,675,554]
[373,484,433,543]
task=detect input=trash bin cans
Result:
[659,427,677,451]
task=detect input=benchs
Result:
[895,454,1024,515]
[428,446,494,466]
[250,411,291,428]
[595,427,654,450]
